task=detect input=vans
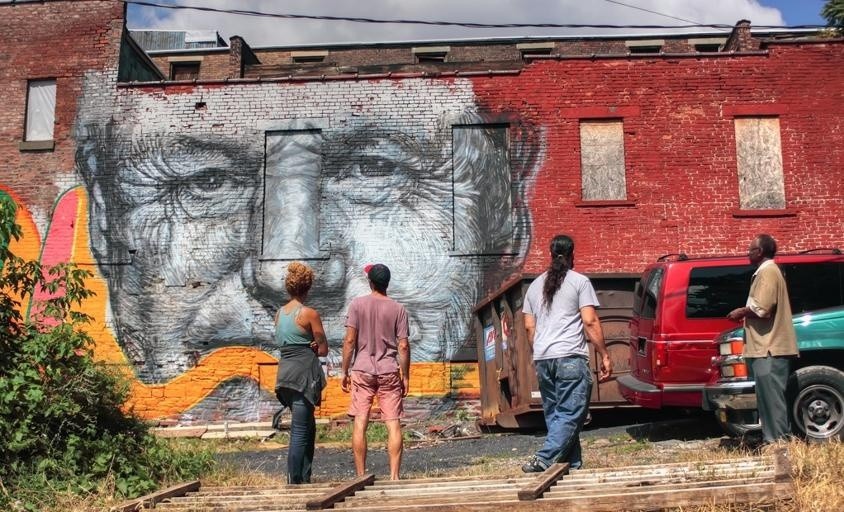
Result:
[615,246,844,413]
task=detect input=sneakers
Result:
[521,461,542,473]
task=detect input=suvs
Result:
[702,305,844,443]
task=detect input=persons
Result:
[270,260,329,487]
[520,234,612,474]
[72,67,540,426]
[725,234,800,449]
[340,262,411,483]
